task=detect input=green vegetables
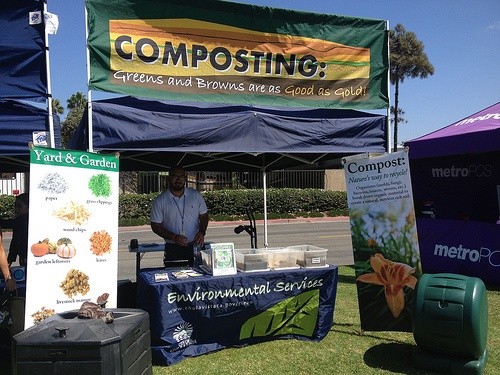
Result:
[88,173,111,198]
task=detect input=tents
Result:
[403,102,500,290]
[0,92,391,247]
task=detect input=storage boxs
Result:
[200,244,329,275]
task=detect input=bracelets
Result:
[199,230,206,236]
[172,234,176,240]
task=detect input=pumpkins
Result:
[31,241,49,257]
[47,237,75,258]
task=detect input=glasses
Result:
[170,175,186,179]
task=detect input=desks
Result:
[128,242,211,270]
[138,263,338,367]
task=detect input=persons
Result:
[150,164,209,268]
[0,193,29,268]
[0,229,17,329]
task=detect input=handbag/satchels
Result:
[0,287,25,344]
